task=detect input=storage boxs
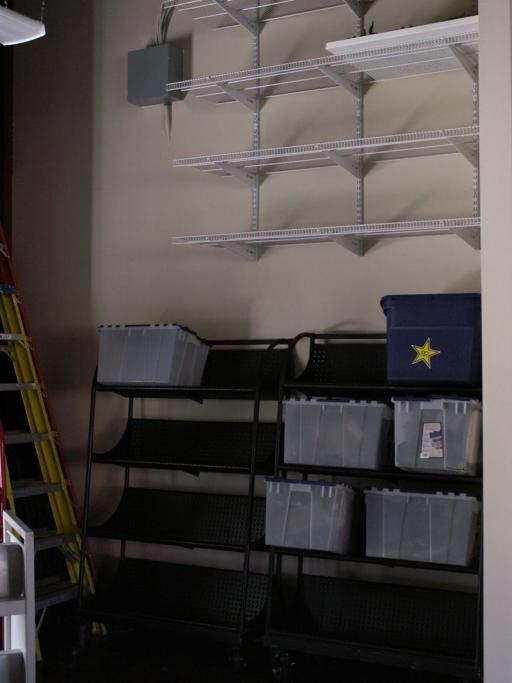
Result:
[280,393,386,472]
[263,478,352,565]
[364,477,481,577]
[391,392,487,480]
[388,295,481,379]
[92,318,213,396]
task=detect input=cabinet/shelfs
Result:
[285,328,484,683]
[166,2,485,261]
[84,316,284,680]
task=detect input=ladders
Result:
[0,222,107,662]
